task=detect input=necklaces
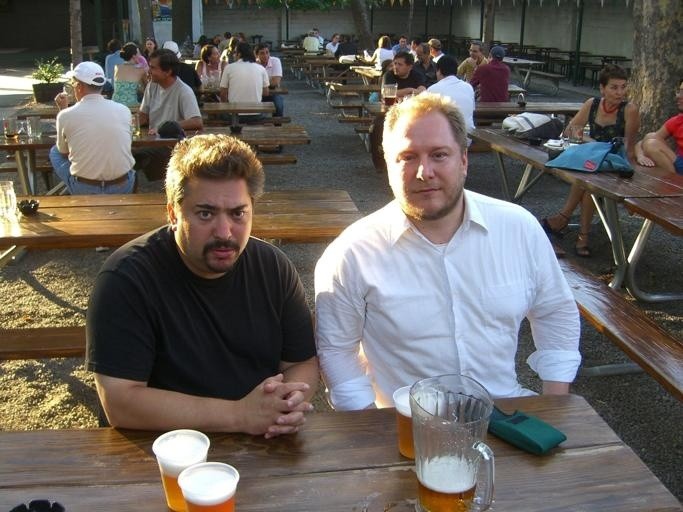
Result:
[122,60,135,65]
[601,97,620,116]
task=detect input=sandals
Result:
[541,213,568,234]
[575,231,590,257]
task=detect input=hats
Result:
[428,38,441,49]
[162,41,182,59]
[491,46,505,58]
[157,120,186,139]
[73,61,107,86]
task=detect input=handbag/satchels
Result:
[503,111,563,139]
[545,137,634,178]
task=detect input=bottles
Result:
[582,123,590,139]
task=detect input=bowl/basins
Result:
[17,199,39,216]
[542,142,578,159]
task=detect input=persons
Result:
[312,93,581,413]
[81,133,320,438]
[633,76,683,174]
[302,28,356,85]
[540,68,639,259]
[48,61,134,195]
[363,35,510,103]
[102,32,285,132]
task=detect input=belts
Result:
[79,176,127,186]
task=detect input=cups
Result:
[611,137,628,160]
[409,373,495,512]
[131,112,141,136]
[392,385,444,459]
[383,83,397,106]
[0,180,17,222]
[150,427,239,512]
[2,114,42,139]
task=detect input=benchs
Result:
[0,312,312,358]
[549,243,681,394]
